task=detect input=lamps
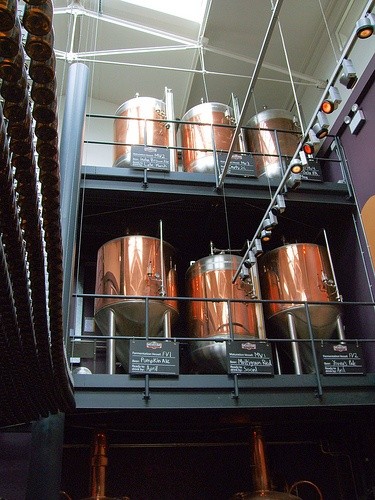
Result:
[300,141,315,155]
[290,158,303,174]
[271,194,286,216]
[239,262,250,281]
[263,210,278,231]
[251,239,263,258]
[342,104,365,136]
[355,11,375,40]
[244,249,256,269]
[338,59,358,90]
[261,227,272,241]
[310,110,329,139]
[322,86,342,114]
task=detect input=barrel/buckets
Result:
[180,102,242,172]
[114,97,170,172]
[244,109,304,180]
